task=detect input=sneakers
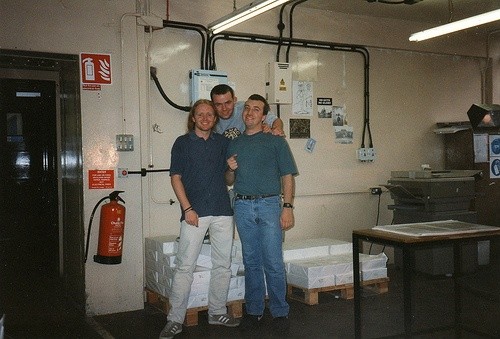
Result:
[277,315,289,331]
[158,321,183,339]
[208,313,241,326]
[240,314,263,330]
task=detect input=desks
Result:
[352,220,500,339]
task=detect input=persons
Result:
[159,100,271,339]
[223,94,297,337]
[177,84,286,244]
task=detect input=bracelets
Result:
[184,206,193,212]
[225,166,235,176]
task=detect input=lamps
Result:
[207,0,289,34]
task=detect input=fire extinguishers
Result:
[97,191,126,265]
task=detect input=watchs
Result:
[283,202,292,208]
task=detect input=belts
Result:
[235,193,279,200]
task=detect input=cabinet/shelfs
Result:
[446,127,500,269]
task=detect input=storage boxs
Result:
[144,234,389,309]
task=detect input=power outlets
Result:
[371,188,381,195]
